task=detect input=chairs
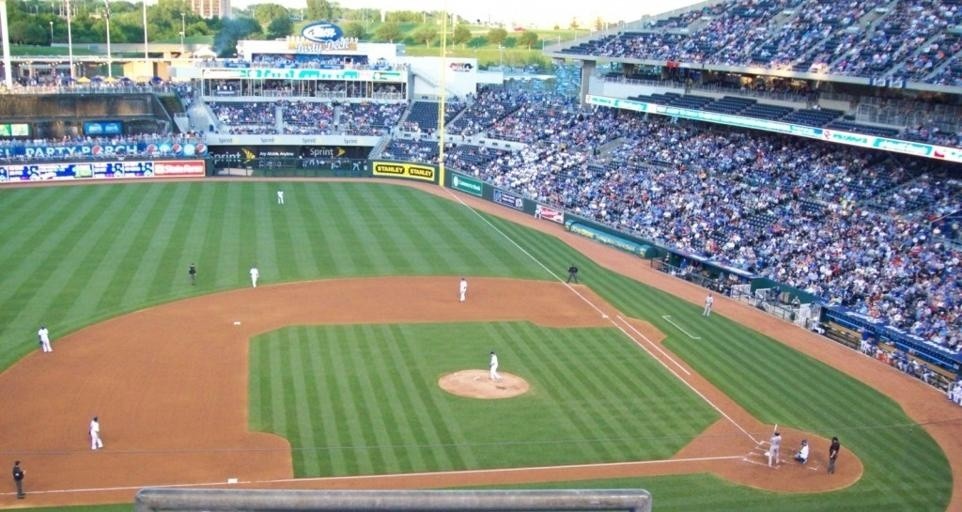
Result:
[391,16,937,251]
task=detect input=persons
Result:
[1,58,411,158]
[87,415,104,450]
[768,431,781,466]
[675,258,962,409]
[189,263,197,285]
[37,324,53,352]
[250,264,260,288]
[794,440,809,464]
[12,460,26,499]
[409,1,960,355]
[826,437,840,474]
[277,189,286,206]
[489,351,502,379]
